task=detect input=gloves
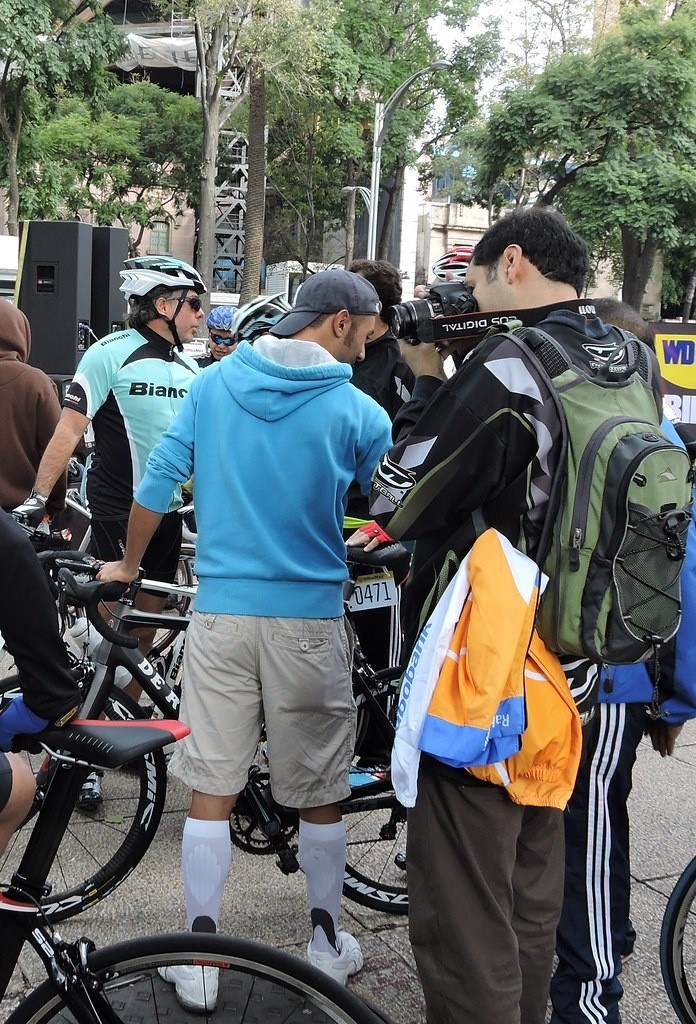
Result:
[10,493,47,537]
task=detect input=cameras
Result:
[387,281,480,340]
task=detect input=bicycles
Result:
[0,719,396,1023]
[659,854,696,1024]
[0,448,408,922]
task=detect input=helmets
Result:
[432,246,475,285]
[117,254,207,302]
[205,305,240,331]
[233,288,293,341]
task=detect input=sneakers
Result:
[75,771,105,813]
[308,931,364,988]
[154,964,221,1014]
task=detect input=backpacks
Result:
[491,326,685,666]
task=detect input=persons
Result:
[547,297,696,1024]
[347,206,662,1024]
[0,298,67,535]
[176,292,292,540]
[11,255,208,774]
[0,506,82,859]
[261,259,417,789]
[96,268,394,1015]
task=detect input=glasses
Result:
[209,332,236,347]
[164,295,204,312]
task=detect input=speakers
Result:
[16,220,93,375]
[90,227,129,346]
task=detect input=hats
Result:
[269,268,379,337]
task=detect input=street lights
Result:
[342,58,454,261]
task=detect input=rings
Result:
[372,529,380,535]
